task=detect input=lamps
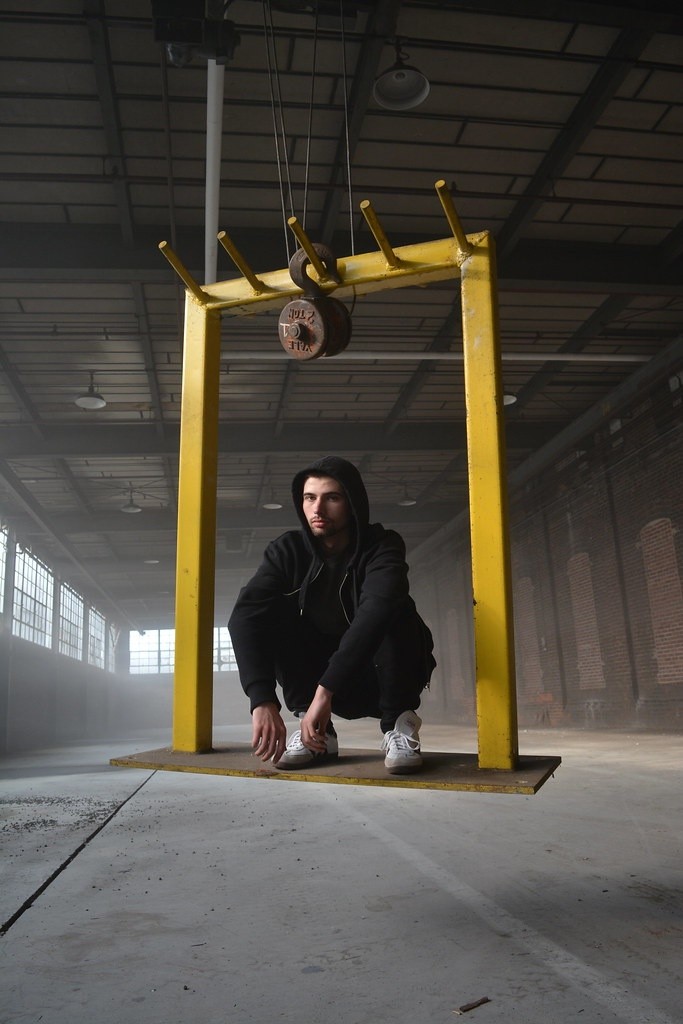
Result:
[144,545,159,563]
[400,503,416,506]
[121,488,142,513]
[74,372,106,409]
[262,486,282,509]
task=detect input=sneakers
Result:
[271,728,338,770]
[380,710,423,774]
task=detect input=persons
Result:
[228,456,437,774]
